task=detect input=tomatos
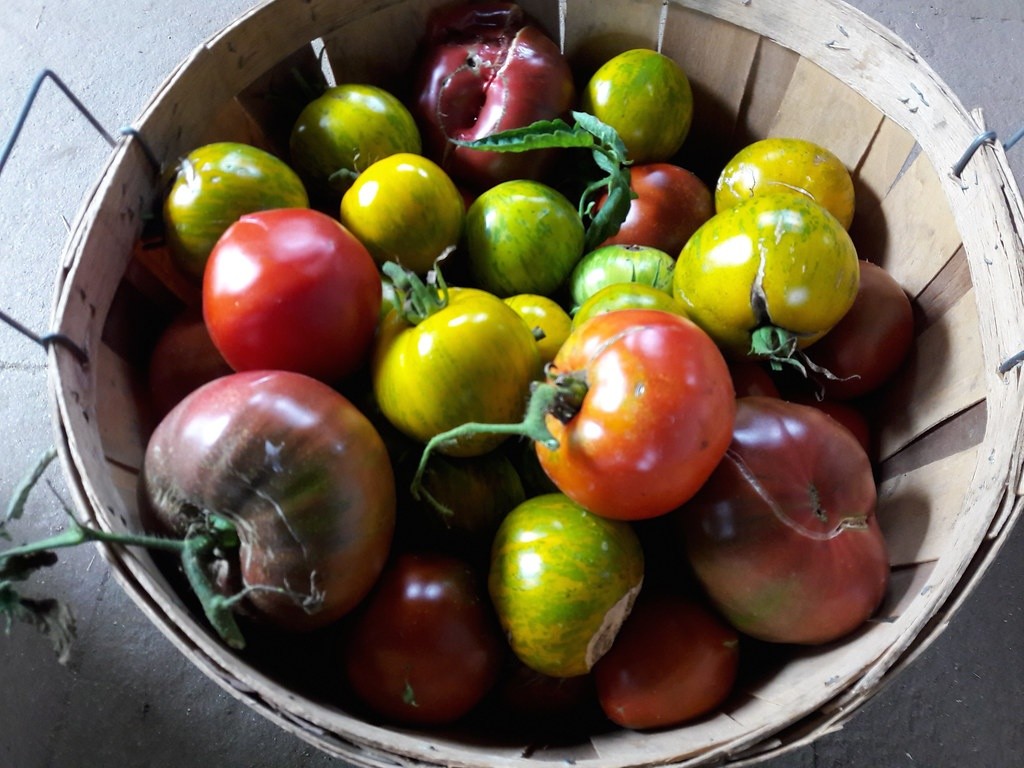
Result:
[144,0,916,729]
